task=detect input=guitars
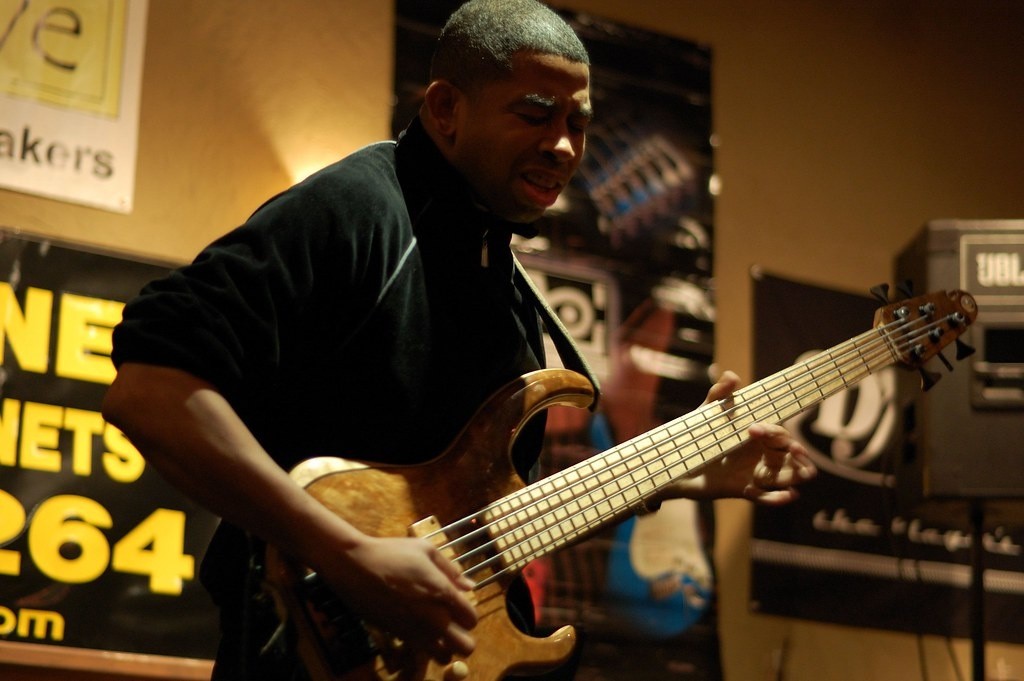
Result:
[193,281,980,681]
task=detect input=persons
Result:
[103,1,815,680]
